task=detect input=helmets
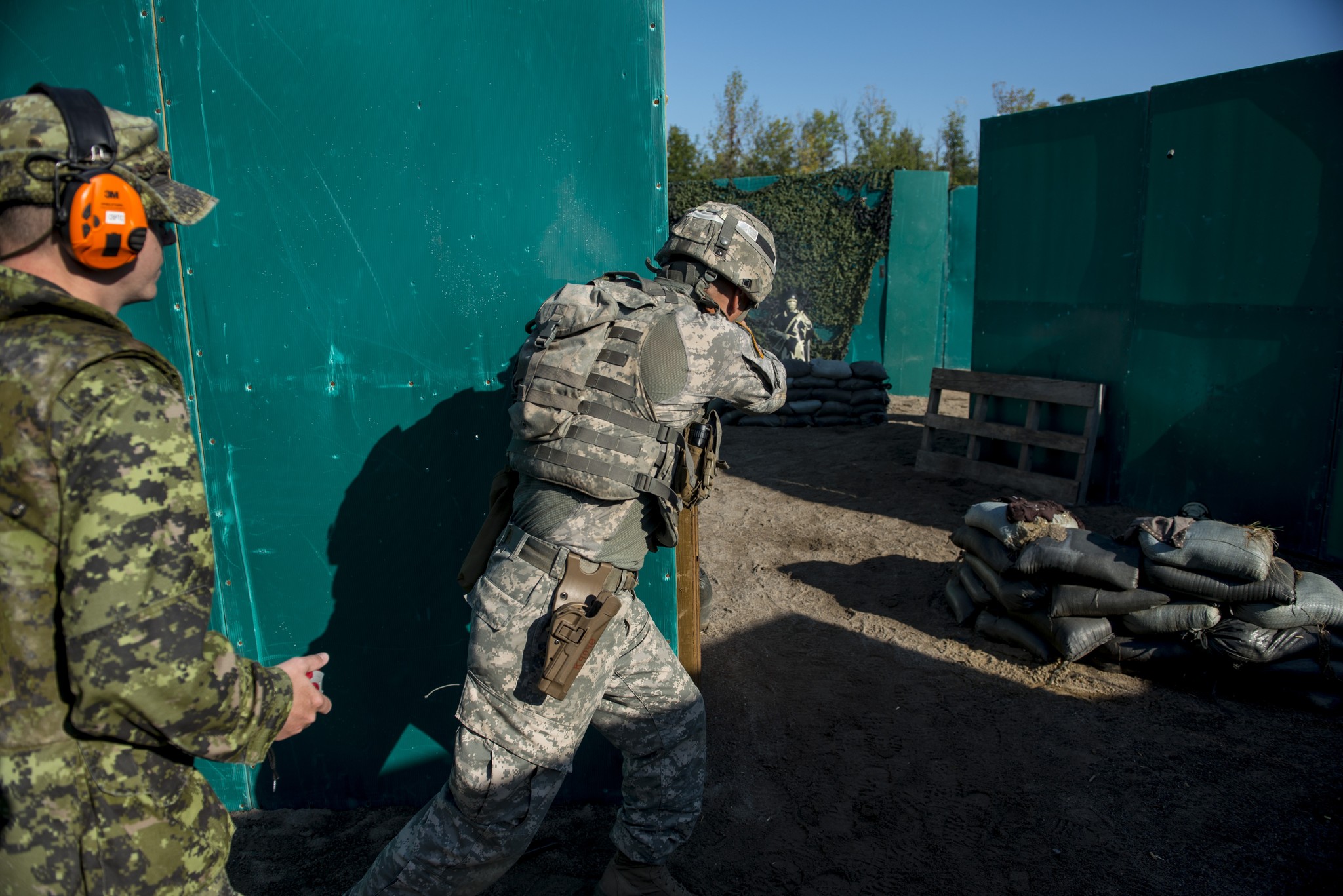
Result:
[654,202,780,306]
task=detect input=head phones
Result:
[26,82,149,270]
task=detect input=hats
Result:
[0,98,218,224]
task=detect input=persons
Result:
[768,287,814,363]
[335,201,791,896]
[1,82,332,896]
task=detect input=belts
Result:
[497,525,640,597]
